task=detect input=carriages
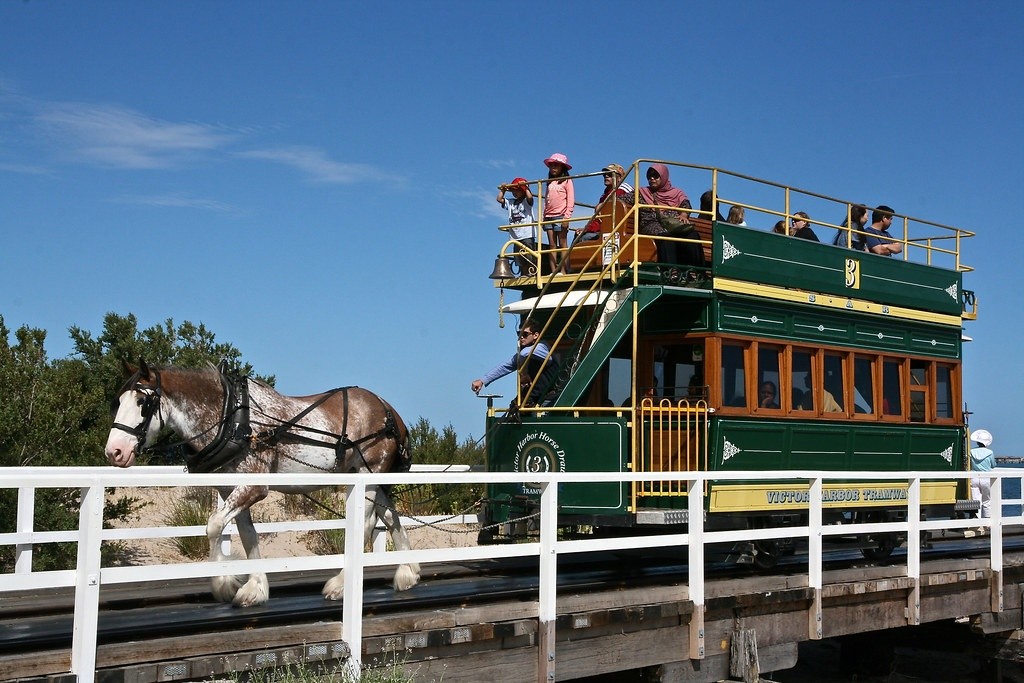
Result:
[104,157,976,606]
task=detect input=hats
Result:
[508,178,529,190]
[971,429,993,446]
[544,153,572,170]
[602,163,624,177]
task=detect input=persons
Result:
[595,163,707,280]
[834,204,869,252]
[497,178,537,276]
[792,371,843,412]
[622,376,658,407]
[471,319,560,408]
[543,153,575,275]
[970,430,997,531]
[864,205,902,257]
[775,221,794,236]
[687,374,704,395]
[698,192,725,222]
[726,205,746,226]
[573,163,635,244]
[759,381,779,408]
[792,212,820,242]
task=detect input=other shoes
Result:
[547,272,564,277]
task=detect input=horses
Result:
[104,356,422,608]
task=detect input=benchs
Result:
[567,200,713,271]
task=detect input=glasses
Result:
[647,172,659,179]
[603,173,612,177]
[516,331,533,337]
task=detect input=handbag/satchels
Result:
[659,213,695,235]
